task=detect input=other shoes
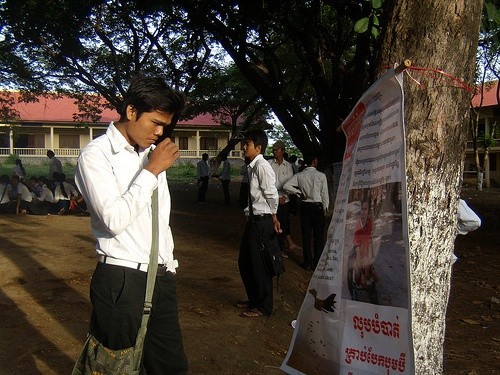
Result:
[197,201,209,206]
[303,262,318,271]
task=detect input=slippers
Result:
[239,309,267,318]
[237,302,253,309]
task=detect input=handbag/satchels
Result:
[254,234,286,278]
[70,325,150,375]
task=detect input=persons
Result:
[352,192,383,305]
[197,140,330,271]
[0,150,87,217]
[75,77,188,375]
[451,198,481,266]
[238,129,283,318]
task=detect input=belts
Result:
[247,213,275,221]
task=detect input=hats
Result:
[269,140,285,151]
